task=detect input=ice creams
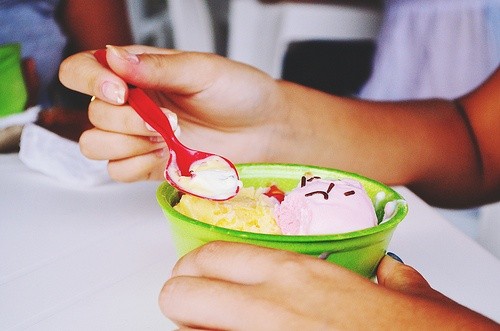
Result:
[171,171,380,236]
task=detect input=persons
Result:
[1,0,133,154]
[58,44,500,331]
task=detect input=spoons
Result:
[93,48,240,201]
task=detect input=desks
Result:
[1,153,499,330]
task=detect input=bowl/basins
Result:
[156,162,408,282]
